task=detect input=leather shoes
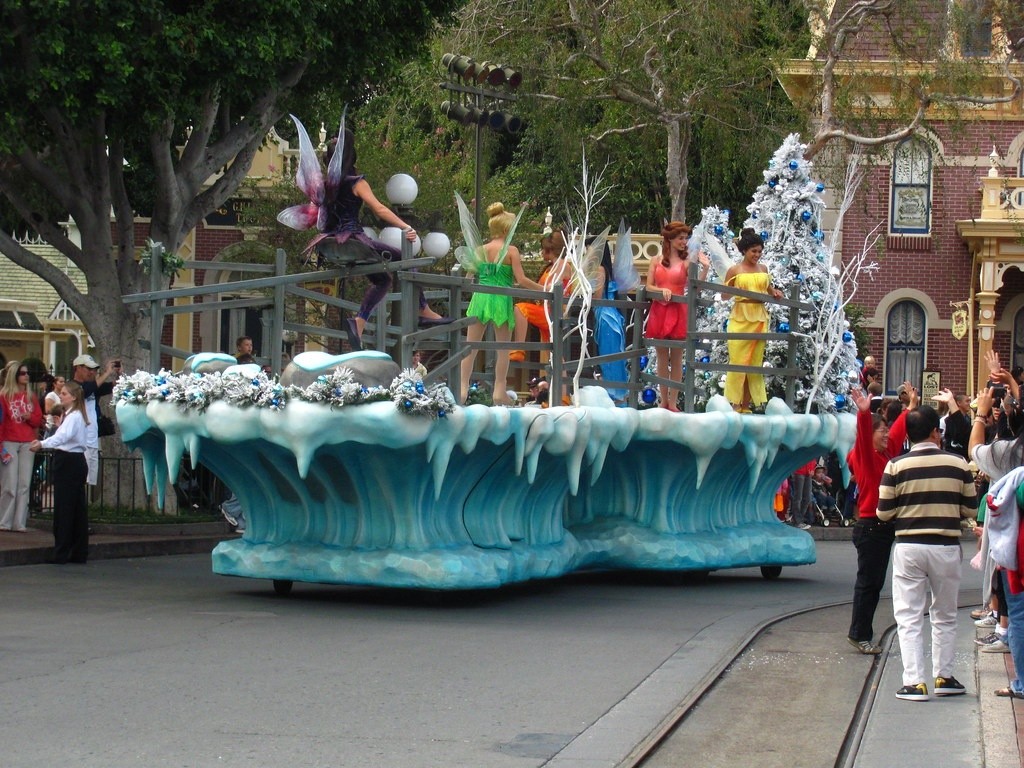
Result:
[418,316,457,328]
[345,318,361,351]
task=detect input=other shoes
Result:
[493,390,512,406]
[969,609,1011,653]
[657,401,680,412]
[509,350,525,363]
[460,386,468,403]
[542,396,570,408]
[994,686,1024,699]
[796,521,812,530]
[18,527,27,532]
[219,504,246,533]
[733,406,752,414]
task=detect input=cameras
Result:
[992,387,1007,398]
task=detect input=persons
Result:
[509,232,571,408]
[777,451,856,529]
[29,381,89,565]
[860,356,910,425]
[218,492,246,533]
[583,236,627,407]
[720,227,783,414]
[461,202,547,405]
[877,405,978,700]
[526,377,548,404]
[932,351,1024,699]
[414,350,427,376]
[645,222,709,412]
[231,336,271,373]
[845,382,918,654]
[0,355,123,534]
[319,138,457,349]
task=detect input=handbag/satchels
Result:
[97,415,116,438]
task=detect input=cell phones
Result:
[113,361,122,368]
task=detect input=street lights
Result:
[440,52,524,242]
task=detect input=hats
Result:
[73,355,100,369]
[814,464,826,470]
[896,384,914,395]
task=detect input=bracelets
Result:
[406,226,411,229]
[975,415,986,420]
[975,419,985,424]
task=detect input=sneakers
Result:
[848,637,881,654]
[934,675,965,693]
[894,683,929,700]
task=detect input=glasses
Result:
[17,371,31,376]
[874,429,890,435]
